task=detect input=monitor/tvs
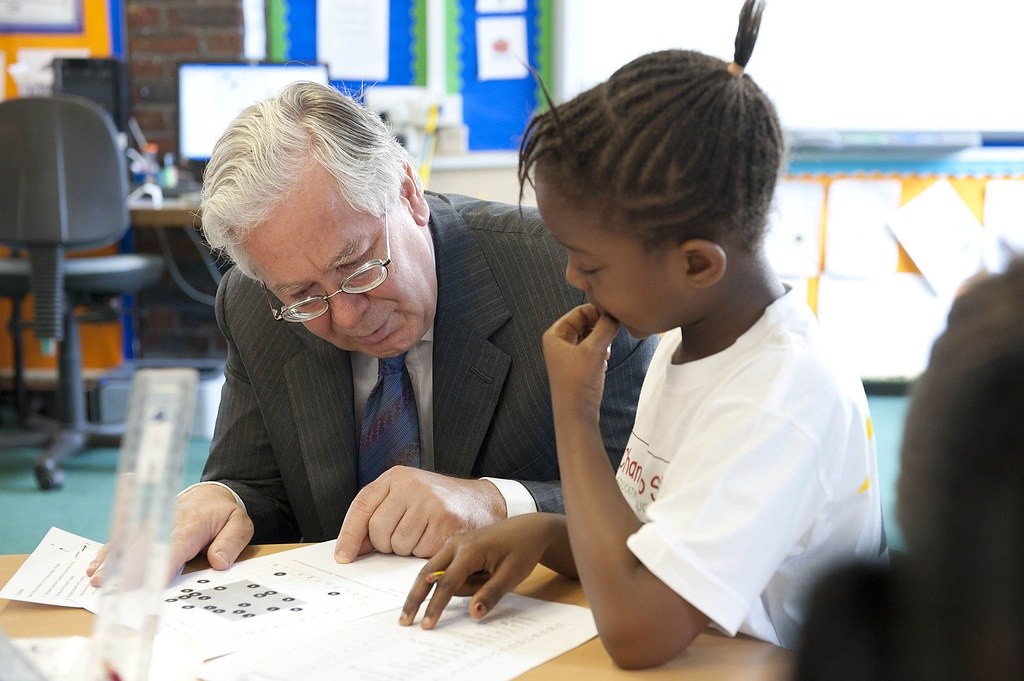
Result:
[176,61,330,180]
[51,60,124,134]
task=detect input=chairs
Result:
[0,97,131,490]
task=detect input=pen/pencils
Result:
[426,570,446,585]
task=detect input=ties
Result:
[357,350,422,492]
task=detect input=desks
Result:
[417,152,538,210]
[130,187,205,227]
[0,541,803,681]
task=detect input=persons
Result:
[399,0,889,669]
[87,79,658,599]
[787,257,1024,681]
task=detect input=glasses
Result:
[260,189,392,323]
